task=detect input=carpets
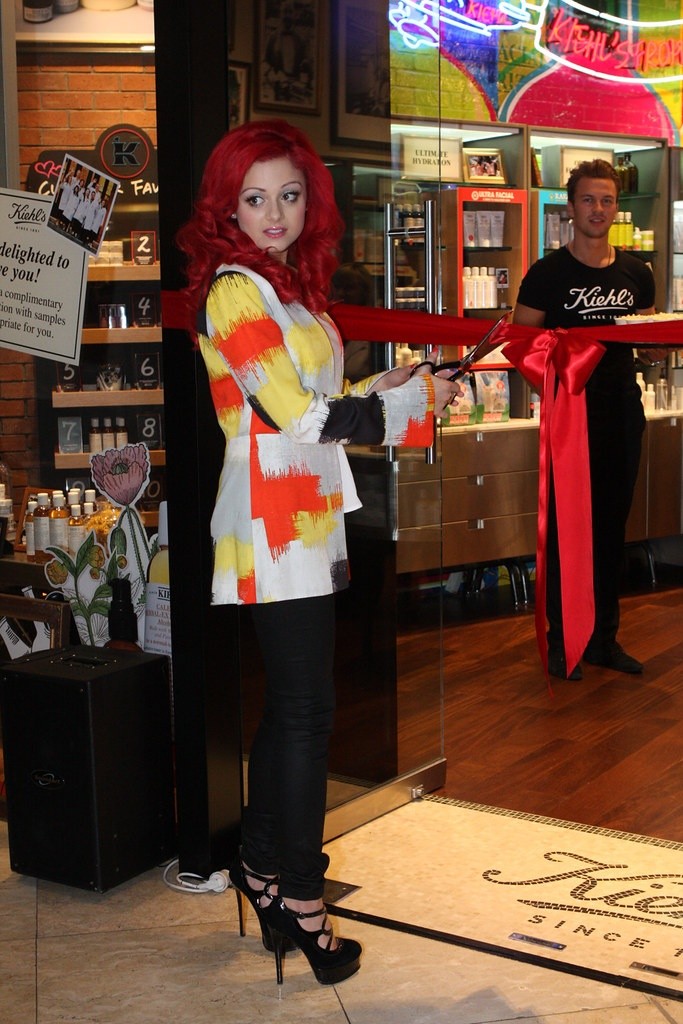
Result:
[321,799,683,1003]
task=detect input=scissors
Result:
[410,310,514,409]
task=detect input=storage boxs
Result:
[132,352,160,390]
[55,347,83,392]
[134,412,160,450]
[130,292,158,328]
[138,469,164,511]
[57,416,83,454]
[64,477,91,498]
[130,231,156,266]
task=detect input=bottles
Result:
[613,151,638,195]
[24,487,99,565]
[393,203,424,228]
[656,373,668,415]
[395,342,422,369]
[636,372,646,406]
[644,384,656,416]
[88,416,128,453]
[608,211,654,251]
[462,266,498,309]
[142,501,171,657]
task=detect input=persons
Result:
[512,158,683,680]
[176,119,467,983]
[58,170,109,245]
[471,155,501,177]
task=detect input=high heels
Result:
[256,876,361,984]
[228,845,296,951]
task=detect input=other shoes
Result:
[546,654,583,680]
[584,645,644,672]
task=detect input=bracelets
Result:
[650,361,661,366]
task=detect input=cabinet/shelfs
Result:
[52,262,162,526]
[396,416,683,613]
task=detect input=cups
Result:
[95,360,126,392]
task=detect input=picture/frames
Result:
[254,0,324,116]
[530,146,543,187]
[461,148,508,184]
[329,0,416,154]
[399,132,463,183]
[228,60,253,131]
[12,486,59,552]
